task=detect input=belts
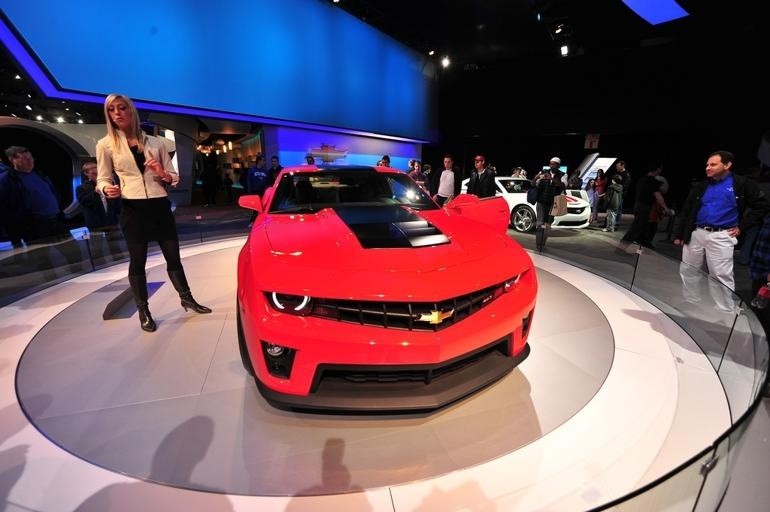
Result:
[697,225,732,231]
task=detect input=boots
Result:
[128,273,156,331]
[166,265,212,313]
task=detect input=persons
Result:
[75,161,124,267]
[199,151,219,208]
[638,155,770,257]
[513,156,564,252]
[0,144,83,283]
[670,151,770,319]
[568,160,630,226]
[266,157,284,187]
[247,155,269,229]
[376,154,495,209]
[223,173,236,204]
[215,167,225,192]
[306,156,314,165]
[95,93,212,333]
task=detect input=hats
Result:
[550,157,561,165]
[473,155,485,160]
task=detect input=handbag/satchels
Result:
[527,187,540,205]
[548,195,567,216]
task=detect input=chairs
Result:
[286,179,322,205]
[359,173,393,202]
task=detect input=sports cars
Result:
[238,162,537,414]
[448,153,617,237]
